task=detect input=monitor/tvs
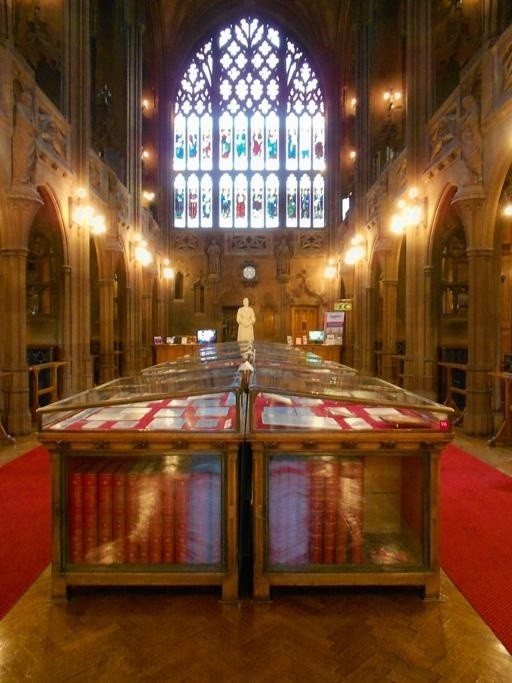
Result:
[196,328,217,344]
[308,330,325,344]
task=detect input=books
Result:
[81,391,237,432]
[262,393,432,431]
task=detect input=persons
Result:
[235,296,256,341]
[205,237,221,273]
[274,238,290,273]
